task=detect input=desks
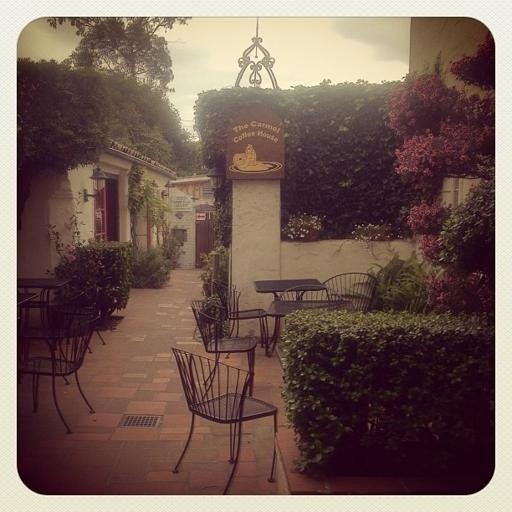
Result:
[18,278,76,299]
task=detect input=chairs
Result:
[18,299,101,434]
[64,275,119,353]
[212,272,377,358]
[191,301,258,385]
[171,348,278,494]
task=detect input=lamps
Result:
[84,163,108,201]
